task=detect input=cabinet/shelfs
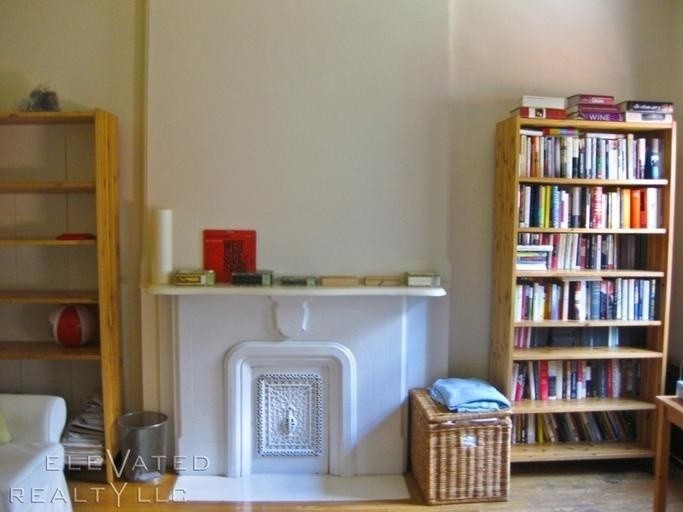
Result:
[0,108,126,486]
[490,114,676,466]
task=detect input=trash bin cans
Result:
[116,412,168,482]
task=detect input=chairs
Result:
[0,393,72,512]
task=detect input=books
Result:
[510,94,675,445]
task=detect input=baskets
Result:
[408,387,513,506]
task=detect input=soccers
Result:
[49,303,95,349]
